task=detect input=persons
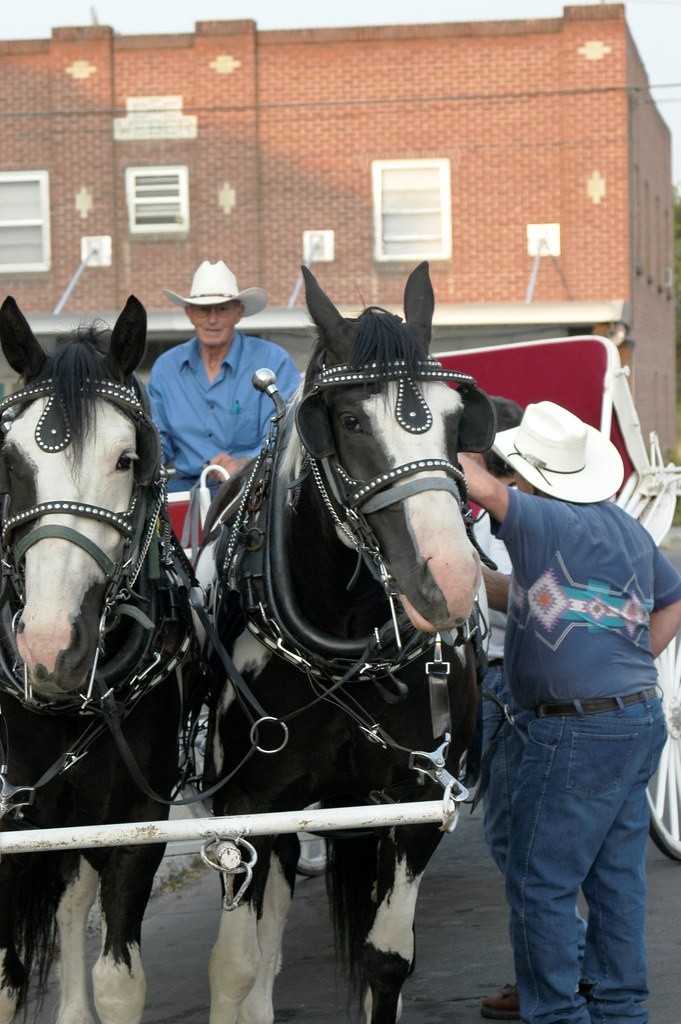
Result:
[145,260,304,493]
[457,397,681,1024]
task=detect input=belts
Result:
[534,688,656,718]
[485,659,503,667]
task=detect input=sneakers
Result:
[480,975,593,1019]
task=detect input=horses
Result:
[1,302,194,1024]
[180,263,492,1024]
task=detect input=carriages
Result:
[0,256,680,1024]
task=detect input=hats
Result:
[162,261,268,317]
[491,401,624,503]
